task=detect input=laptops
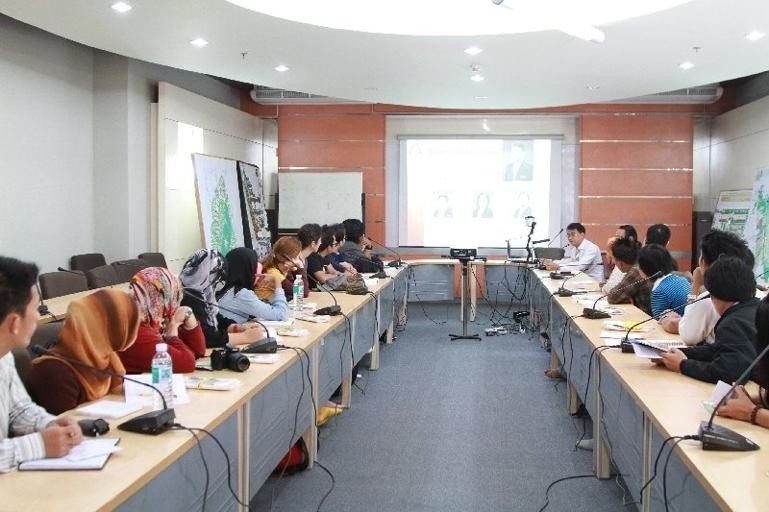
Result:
[506,239,528,261]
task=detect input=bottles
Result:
[293,272,306,312]
[152,343,175,411]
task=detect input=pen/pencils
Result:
[296,318,317,323]
[614,324,625,328]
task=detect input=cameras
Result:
[210,343,250,372]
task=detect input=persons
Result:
[0,255,86,473]
[261,218,384,421]
[539,224,604,337]
[25,288,143,418]
[544,224,768,449]
[117,248,287,374]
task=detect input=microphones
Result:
[367,236,402,267]
[583,269,662,319]
[182,289,276,352]
[557,268,589,296]
[534,228,564,269]
[620,294,708,355]
[345,253,389,277]
[700,344,760,451]
[29,343,175,434]
[280,252,340,315]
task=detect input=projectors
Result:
[451,248,477,259]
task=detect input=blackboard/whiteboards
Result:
[277,172,363,236]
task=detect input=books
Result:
[78,398,142,421]
[18,434,122,472]
